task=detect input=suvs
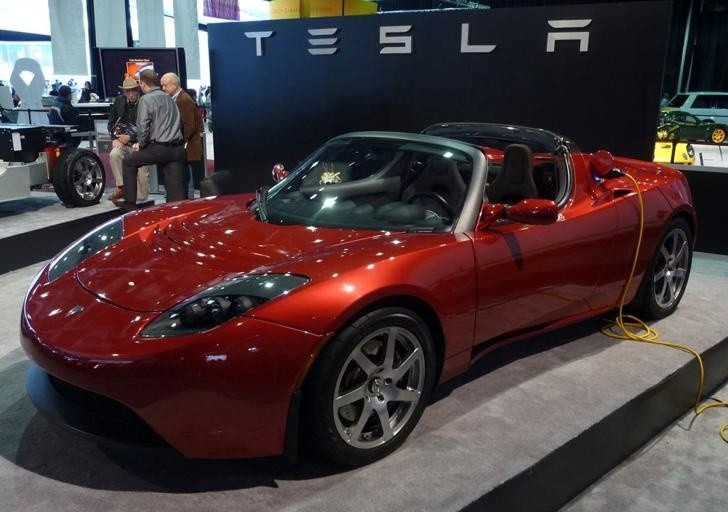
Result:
[659,91,727,124]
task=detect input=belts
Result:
[149,140,169,145]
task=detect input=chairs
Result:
[397,151,467,221]
[484,144,539,204]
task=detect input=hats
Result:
[117,78,140,90]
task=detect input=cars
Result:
[0,103,105,210]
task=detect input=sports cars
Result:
[19,121,697,465]
[655,110,727,146]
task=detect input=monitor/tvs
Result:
[93,47,186,102]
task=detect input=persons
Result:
[0,79,213,148]
[106,78,150,204]
[158,72,205,203]
[111,69,191,212]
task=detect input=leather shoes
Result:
[112,199,137,212]
[107,189,124,199]
